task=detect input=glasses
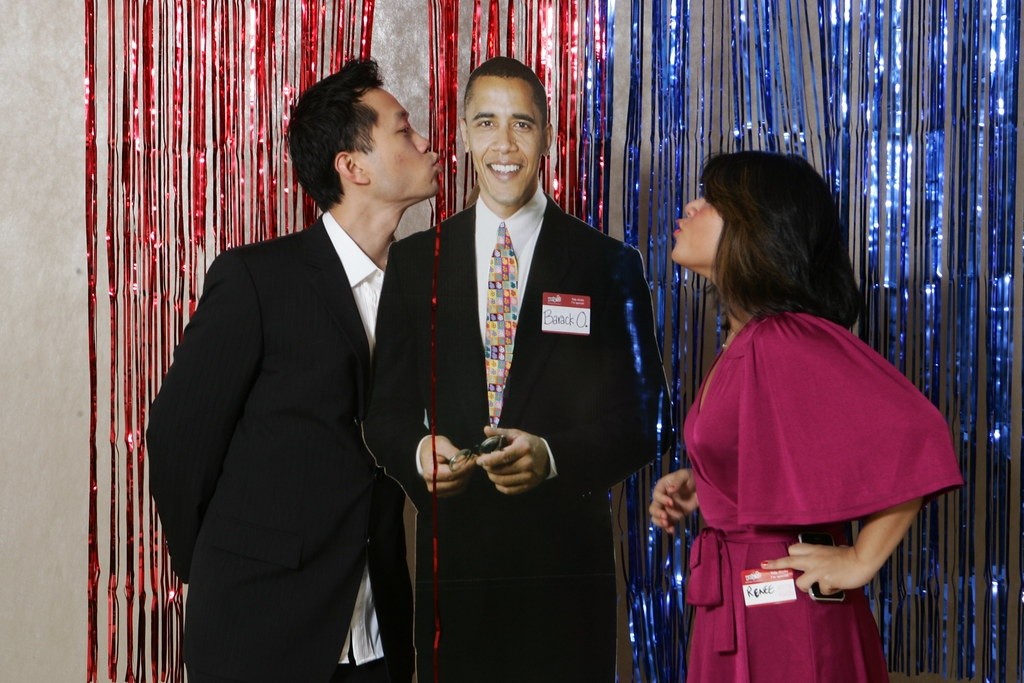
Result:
[448,435,505,472]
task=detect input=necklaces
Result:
[721,316,750,349]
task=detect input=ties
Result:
[484,222,517,431]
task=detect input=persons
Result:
[362,56,671,683]
[649,150,965,682]
[149,56,443,683]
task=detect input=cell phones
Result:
[799,531,846,602]
